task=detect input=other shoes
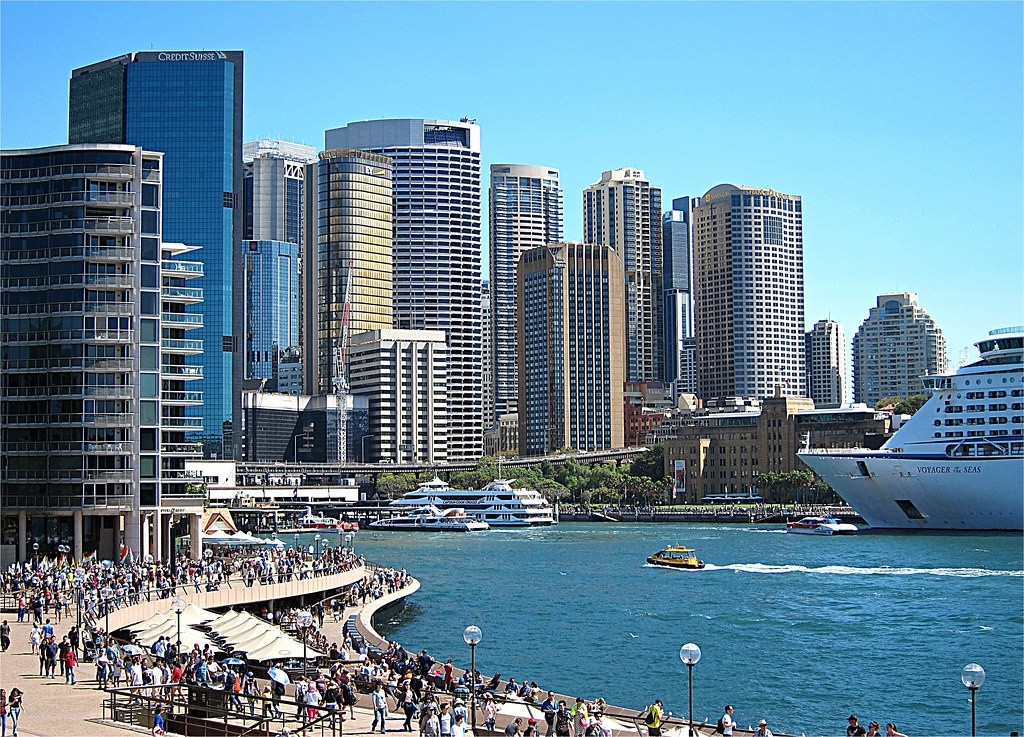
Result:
[409,728,412,733]
[372,726,376,731]
[403,724,407,731]
[381,730,385,734]
[13,733,17,737]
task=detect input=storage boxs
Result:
[307,555,312,561]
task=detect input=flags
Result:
[121,546,132,564]
[86,551,95,561]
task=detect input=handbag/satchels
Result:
[580,713,588,726]
[489,718,495,724]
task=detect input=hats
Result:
[156,704,165,710]
[453,698,464,705]
[459,678,465,683]
[307,683,316,692]
[846,714,857,720]
[655,699,663,706]
[247,672,253,676]
[483,692,493,698]
[403,679,411,684]
[758,720,768,727]
[588,716,601,725]
[427,706,434,710]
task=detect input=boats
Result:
[296,506,358,534]
[368,505,490,532]
[387,456,558,527]
[647,545,706,569]
[787,517,858,535]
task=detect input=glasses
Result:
[868,726,876,728]
[558,705,562,707]
[426,702,431,703]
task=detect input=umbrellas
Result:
[122,645,142,654]
[222,658,245,665]
[267,667,290,685]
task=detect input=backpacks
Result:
[274,681,286,696]
[717,713,725,733]
[645,710,657,724]
[341,682,358,706]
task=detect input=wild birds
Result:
[560,572,566,576]
[630,633,639,639]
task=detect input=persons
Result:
[846,715,909,737]
[753,719,773,737]
[721,705,737,737]
[646,699,664,737]
[0,544,607,737]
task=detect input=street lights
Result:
[463,625,482,735]
[172,598,185,661]
[680,643,701,737]
[101,560,110,587]
[294,433,306,463]
[297,612,313,676]
[144,555,153,601]
[205,549,214,583]
[961,663,986,737]
[362,435,374,464]
[270,528,355,565]
[100,587,113,635]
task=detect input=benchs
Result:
[346,614,499,695]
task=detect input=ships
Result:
[795,325,1024,532]
